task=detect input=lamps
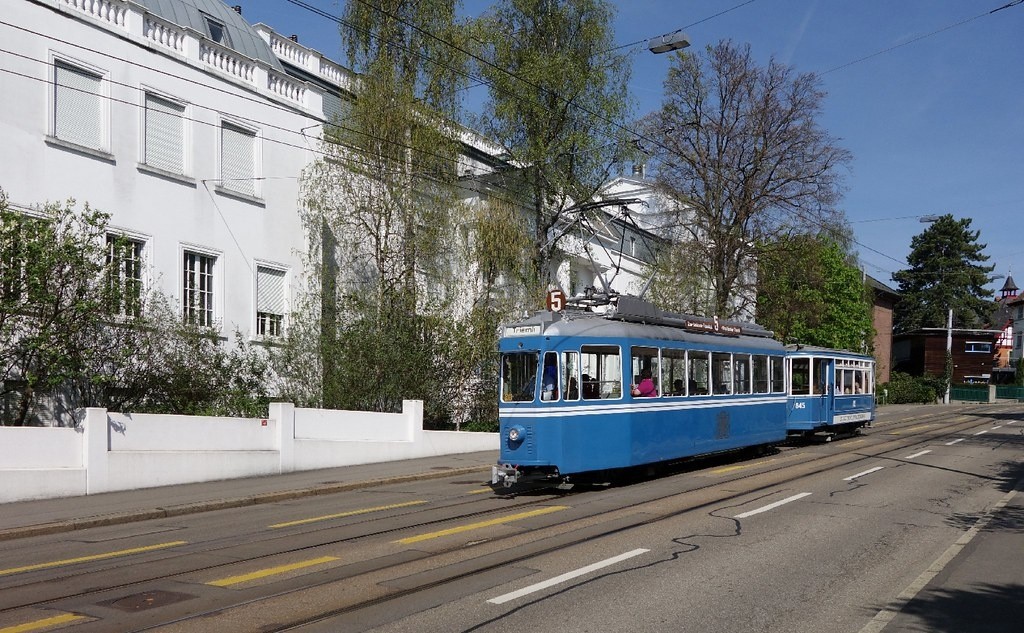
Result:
[647,30,691,54]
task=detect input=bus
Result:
[486,196,877,496]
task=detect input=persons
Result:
[563,377,578,399]
[688,380,698,391]
[590,378,599,398]
[581,374,592,400]
[514,361,555,400]
[713,378,722,394]
[856,382,862,394]
[755,381,766,393]
[672,380,685,396]
[630,370,656,397]
